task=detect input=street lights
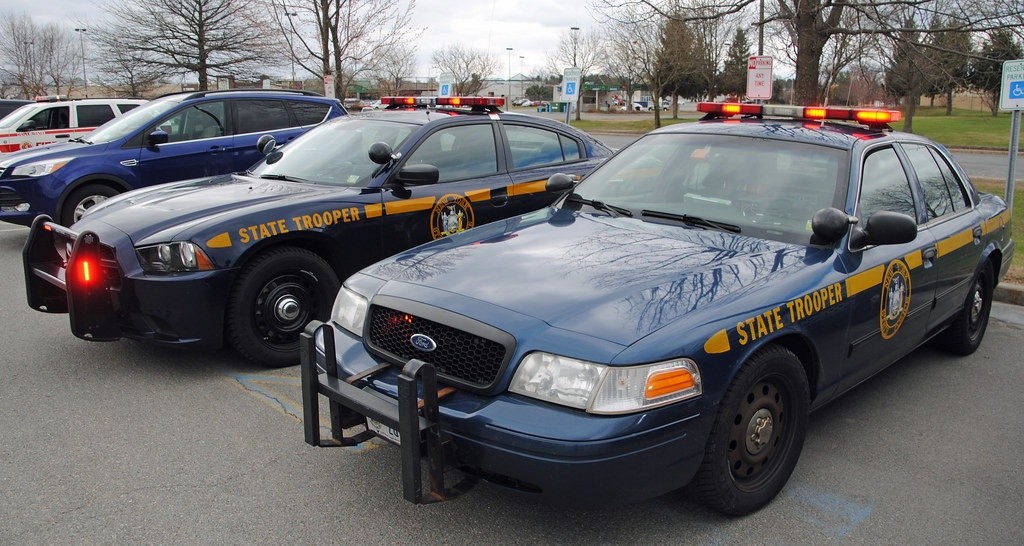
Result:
[76,29,88,98]
[24,41,34,92]
[520,56,525,99]
[507,48,513,100]
[285,12,297,89]
[570,27,580,67]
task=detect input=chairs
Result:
[58,112,68,129]
[186,105,222,140]
[450,132,493,176]
[407,132,443,164]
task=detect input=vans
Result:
[0,96,149,153]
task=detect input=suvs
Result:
[0,89,348,228]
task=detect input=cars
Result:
[22,95,620,370]
[648,101,670,111]
[300,102,1013,517]
[620,103,643,112]
[512,98,551,107]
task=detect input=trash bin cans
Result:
[550,101,570,112]
